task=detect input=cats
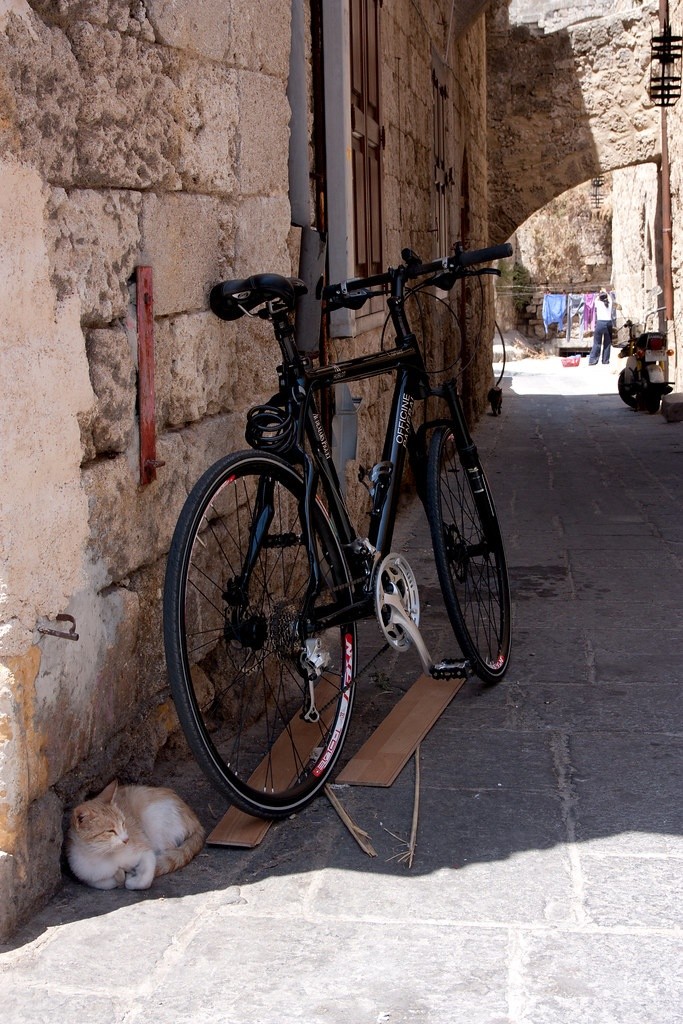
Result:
[67,780,201,891]
[487,387,504,416]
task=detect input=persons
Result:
[588,288,613,365]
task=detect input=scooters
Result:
[610,301,675,414]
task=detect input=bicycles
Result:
[163,238,514,825]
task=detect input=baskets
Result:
[607,317,640,348]
